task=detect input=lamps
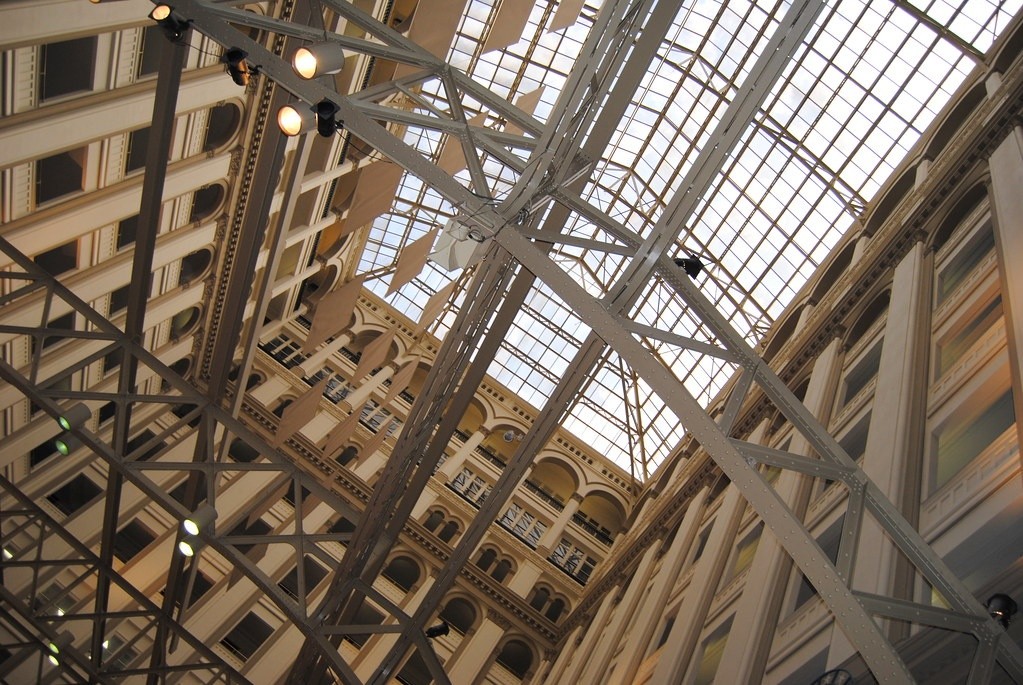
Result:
[184,498,218,535]
[987,593,1019,630]
[278,102,317,136]
[179,533,206,556]
[292,39,344,79]
[55,432,84,455]
[426,621,449,637]
[310,96,345,137]
[49,651,67,666]
[674,254,703,280]
[221,46,259,86]
[50,630,75,654]
[58,402,92,431]
[148,2,190,41]
